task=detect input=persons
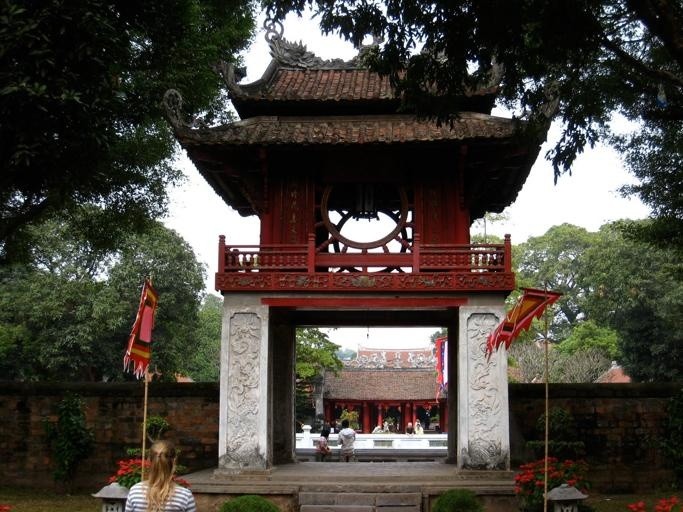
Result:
[414,422,423,434]
[415,418,421,426]
[405,421,414,434]
[337,418,357,462]
[316,429,331,461]
[123,438,196,511]
[424,414,430,429]
[382,416,402,432]
[371,424,382,433]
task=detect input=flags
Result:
[435,337,448,396]
[483,287,563,357]
[120,280,159,380]
